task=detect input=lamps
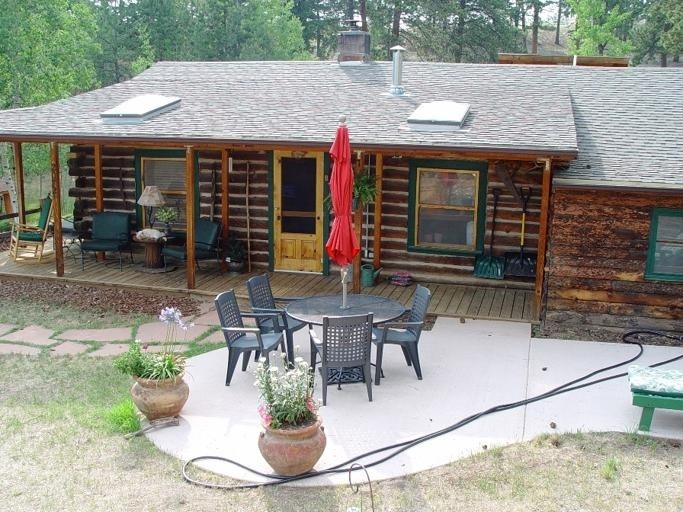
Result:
[137,186,166,229]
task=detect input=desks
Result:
[132,232,178,273]
[285,294,407,378]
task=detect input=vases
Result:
[131,370,189,420]
[257,414,327,476]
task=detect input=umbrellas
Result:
[324,114,361,309]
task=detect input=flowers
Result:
[112,304,197,387]
[246,344,324,428]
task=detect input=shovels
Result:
[503,186,537,277]
[472,187,504,279]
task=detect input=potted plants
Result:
[224,230,245,276]
[155,207,176,234]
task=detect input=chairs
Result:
[371,283,430,383]
[53,209,83,264]
[308,312,372,404]
[161,219,221,272]
[246,273,306,370]
[214,287,287,387]
[79,212,133,272]
[8,193,54,265]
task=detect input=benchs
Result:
[625,363,683,431]
